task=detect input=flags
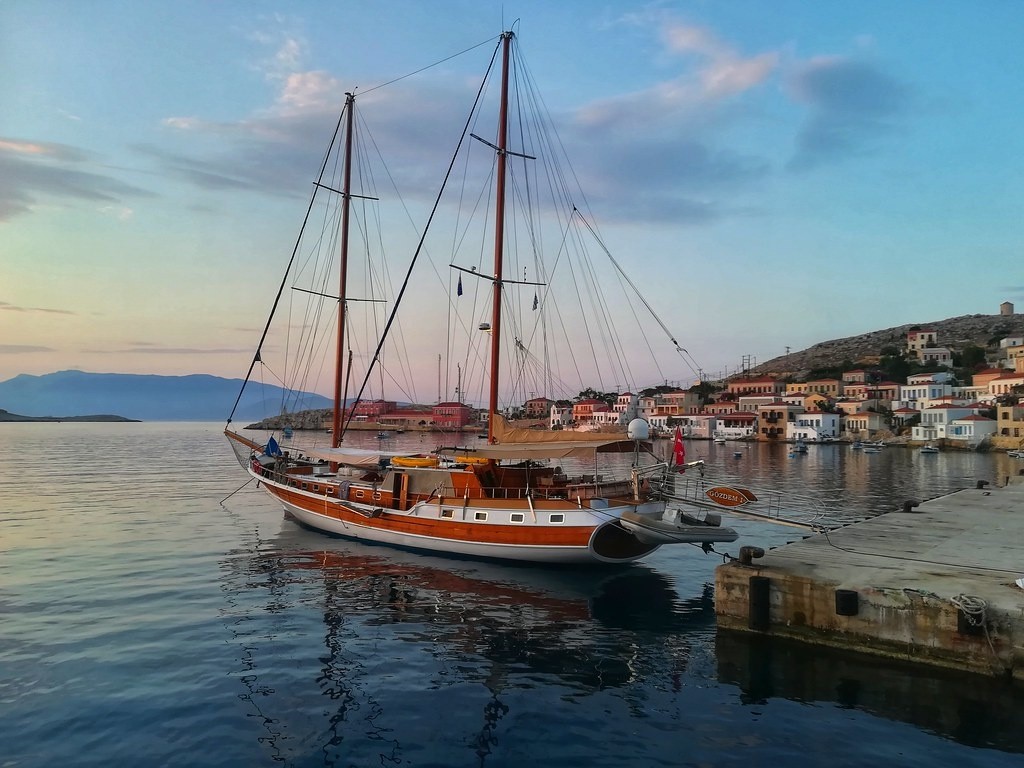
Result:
[673,427,686,474]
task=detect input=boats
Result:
[849,440,887,453]
[1008,449,1024,458]
[919,447,943,454]
[714,438,728,445]
[791,440,807,453]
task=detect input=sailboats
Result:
[224,29,670,564]
